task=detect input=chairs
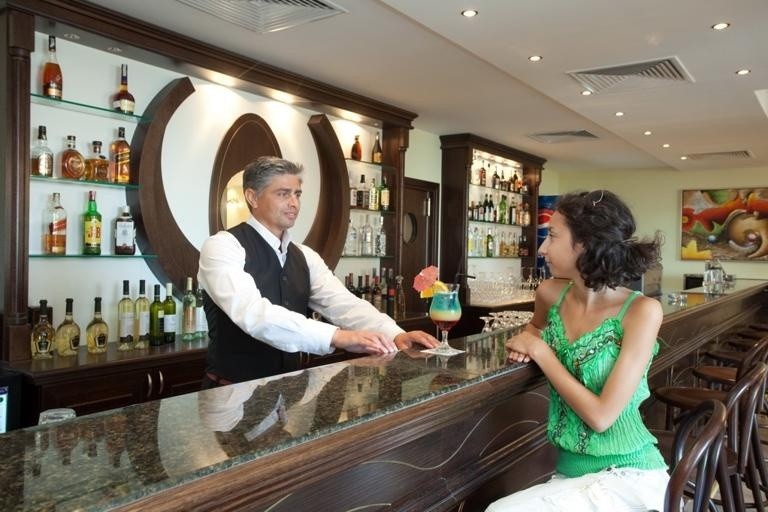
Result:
[648,321,768,512]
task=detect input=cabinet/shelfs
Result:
[439,132,547,309]
[0,0,419,427]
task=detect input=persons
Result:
[195,350,443,459]
[196,155,441,394]
[484,187,683,512]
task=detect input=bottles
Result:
[29,298,57,362]
[23,425,50,480]
[117,277,207,352]
[371,131,384,164]
[346,364,380,422]
[348,171,394,213]
[465,326,535,369]
[77,413,106,458]
[113,205,136,256]
[85,140,109,181]
[40,192,68,257]
[110,126,133,185]
[53,420,80,469]
[30,123,54,180]
[108,63,137,115]
[344,266,407,323]
[55,135,86,180]
[345,214,386,256]
[54,298,82,357]
[468,159,531,258]
[86,296,110,354]
[351,134,363,163]
[42,33,63,101]
[100,407,131,470]
[79,190,103,256]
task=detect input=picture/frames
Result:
[681,186,768,262]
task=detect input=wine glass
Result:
[479,310,534,336]
[419,282,467,357]
[468,266,548,306]
[426,354,468,392]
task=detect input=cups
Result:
[702,256,733,284]
[702,285,728,302]
[679,301,687,310]
[668,302,678,308]
[666,290,680,302]
[679,292,687,301]
